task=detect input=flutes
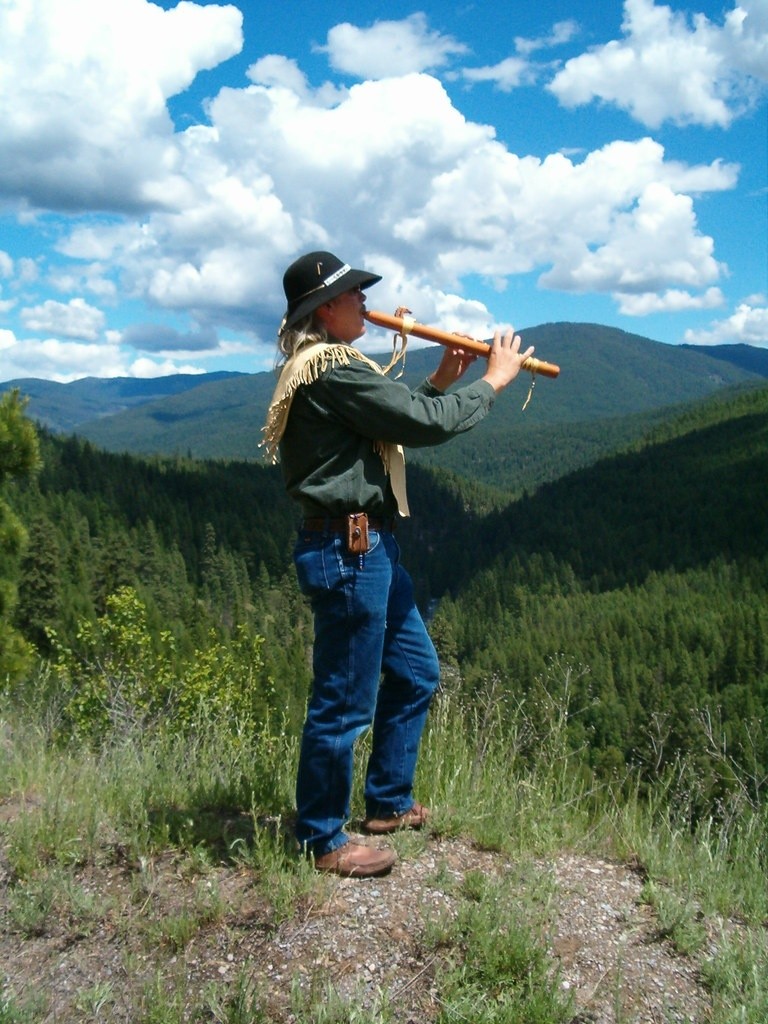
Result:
[362,310,560,378]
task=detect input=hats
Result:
[278,251,382,337]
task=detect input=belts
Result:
[301,518,376,531]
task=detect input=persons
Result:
[259,251,534,877]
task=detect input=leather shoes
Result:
[311,841,397,876]
[362,802,457,832]
